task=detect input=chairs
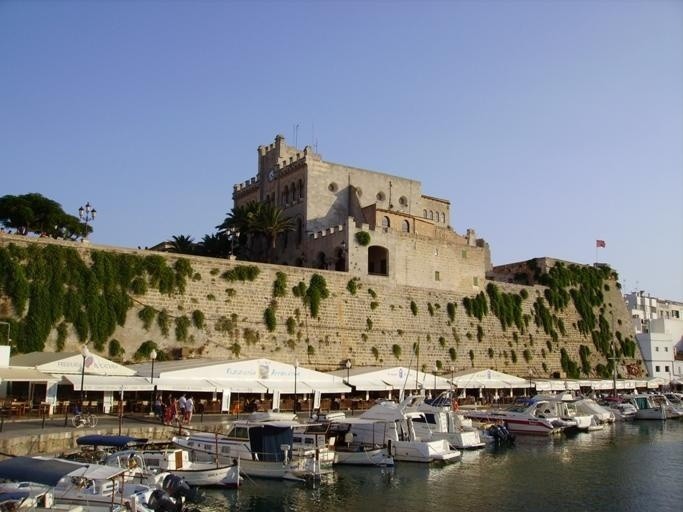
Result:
[0,399,127,417]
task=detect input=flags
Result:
[596,240,605,247]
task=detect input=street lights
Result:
[80,345,89,390]
[528,368,533,387]
[79,202,96,239]
[433,367,438,389]
[226,227,241,255]
[451,365,454,385]
[346,359,352,384]
[150,348,158,383]
[293,358,299,393]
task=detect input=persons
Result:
[76,399,82,415]
[243,397,264,412]
[154,392,195,426]
